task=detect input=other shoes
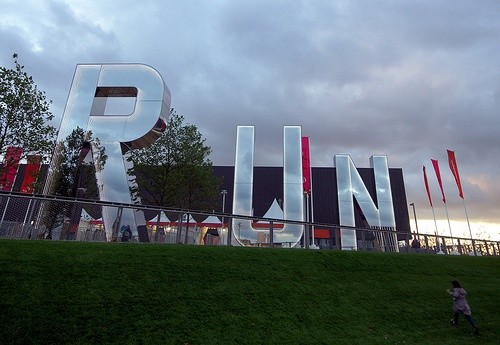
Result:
[472,327,480,336]
[450,318,458,326]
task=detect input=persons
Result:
[446,280,480,334]
